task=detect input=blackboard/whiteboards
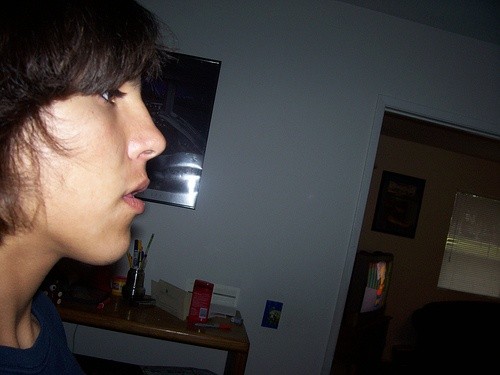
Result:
[436,191,499,298]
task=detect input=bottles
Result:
[127,269,145,301]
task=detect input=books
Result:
[151,279,192,321]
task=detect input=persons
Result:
[0,0,166,375]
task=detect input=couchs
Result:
[391,300,499,375]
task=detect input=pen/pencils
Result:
[126,233,155,269]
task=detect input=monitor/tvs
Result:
[344,250,394,316]
[133,46,222,210]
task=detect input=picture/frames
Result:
[372,170,426,239]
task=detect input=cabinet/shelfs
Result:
[55,289,250,375]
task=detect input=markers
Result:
[97,296,111,311]
[193,321,235,329]
